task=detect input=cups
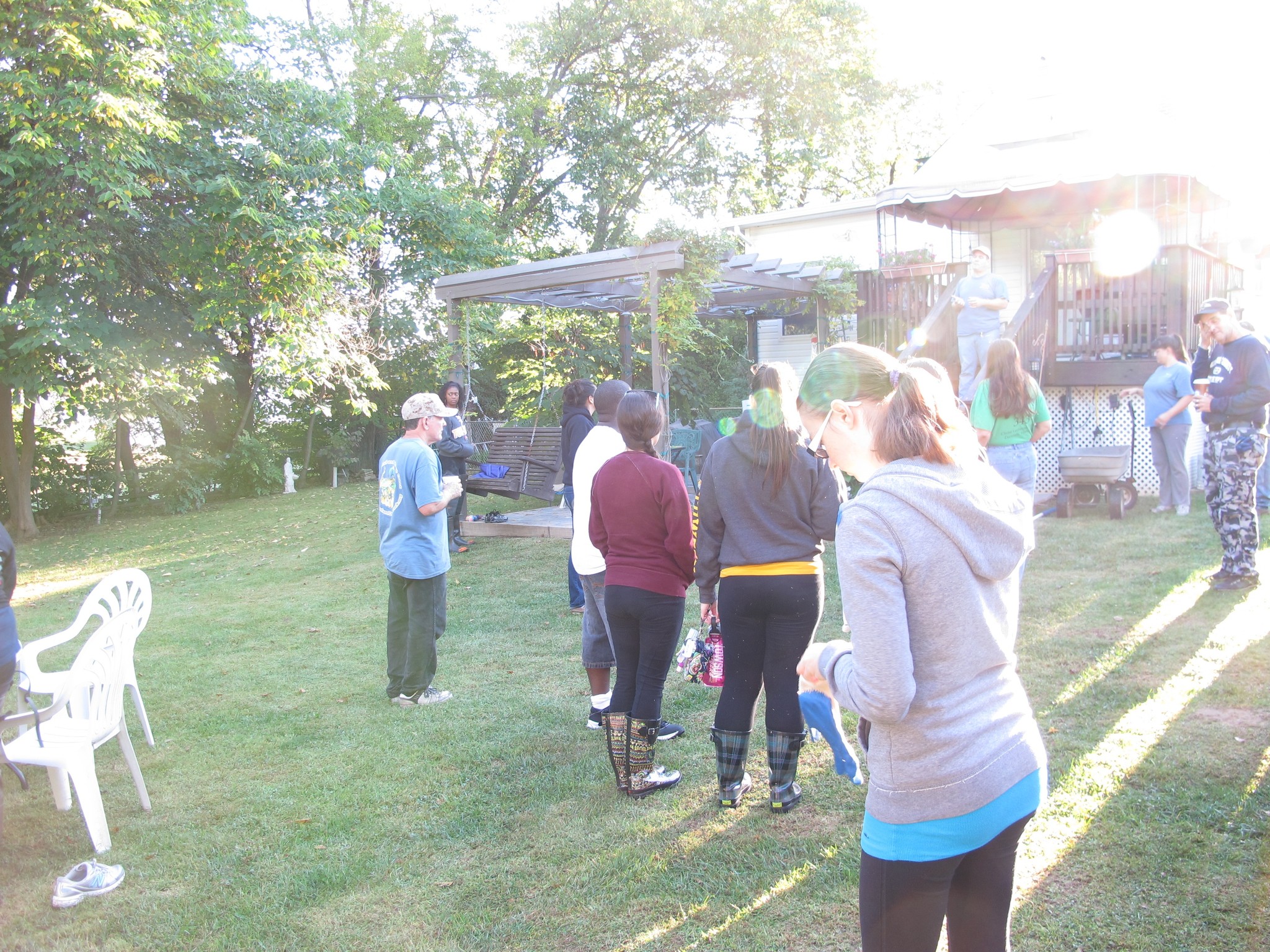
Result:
[1193,379,1211,410]
[442,476,459,485]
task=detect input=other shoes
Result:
[1150,505,1173,512]
[1215,574,1259,590]
[1206,569,1230,583]
[958,404,970,415]
[1176,504,1188,515]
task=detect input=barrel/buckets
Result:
[1095,334,1124,360]
[1196,453,1204,492]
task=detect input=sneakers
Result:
[489,509,508,523]
[389,685,432,703]
[586,707,604,730]
[399,687,452,707]
[52,858,126,907]
[484,509,496,522]
[656,721,685,741]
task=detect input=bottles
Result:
[701,616,724,688]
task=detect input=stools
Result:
[1069,318,1107,360]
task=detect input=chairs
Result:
[0,568,154,855]
[670,429,702,494]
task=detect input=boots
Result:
[453,517,475,546]
[602,710,628,792]
[447,517,469,553]
[623,711,681,799]
[766,727,808,814]
[709,720,752,808]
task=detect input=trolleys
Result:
[1055,392,1138,520]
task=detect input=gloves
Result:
[797,673,863,786]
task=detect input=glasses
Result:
[805,400,862,459]
[625,389,661,410]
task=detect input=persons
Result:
[795,339,1050,952]
[694,361,847,813]
[587,391,696,797]
[377,393,464,707]
[0,519,22,707]
[428,245,1269,616]
[570,379,684,742]
[282,457,297,494]
[432,381,475,553]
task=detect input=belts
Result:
[1205,420,1264,431]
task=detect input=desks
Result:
[671,447,687,464]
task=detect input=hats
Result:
[971,246,990,259]
[401,393,459,420]
[1193,297,1230,325]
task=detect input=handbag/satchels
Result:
[683,616,723,687]
